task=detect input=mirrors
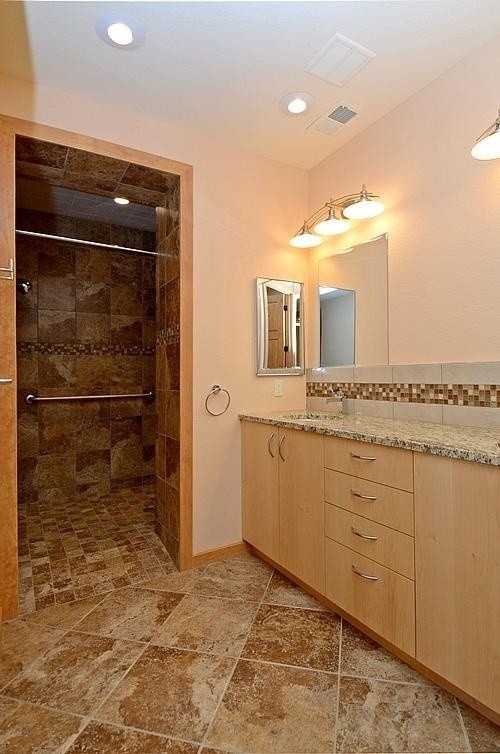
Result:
[317,232,390,367]
[255,275,307,375]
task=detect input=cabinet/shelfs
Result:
[411,450,499,711]
[241,420,326,597]
[320,433,415,661]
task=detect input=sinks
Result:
[283,412,344,421]
[325,389,345,414]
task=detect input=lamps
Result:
[466,109,500,160]
[287,182,386,249]
